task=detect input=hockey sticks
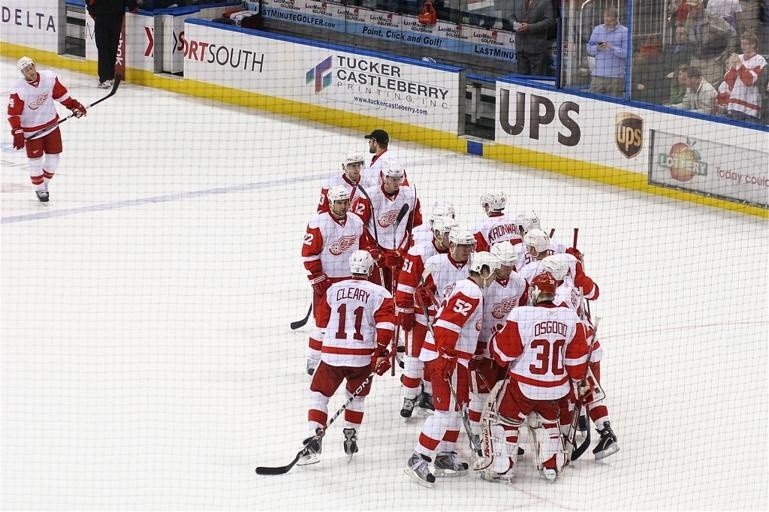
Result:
[565,317,600,469]
[358,185,385,288]
[256,348,397,475]
[392,204,409,377]
[424,304,480,457]
[291,303,312,329]
[570,378,591,460]
[25,73,121,140]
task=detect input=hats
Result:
[364,129,389,147]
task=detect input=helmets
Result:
[15,56,35,77]
[326,153,571,291]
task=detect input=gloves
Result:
[11,127,25,150]
[574,261,596,299]
[65,99,87,119]
[571,378,593,406]
[439,350,458,381]
[370,347,391,376]
[397,307,415,332]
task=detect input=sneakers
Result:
[400,395,418,423]
[418,383,435,415]
[433,451,469,478]
[402,449,436,488]
[579,415,587,438]
[396,346,405,368]
[343,428,359,464]
[540,468,556,482]
[477,439,524,484]
[97,80,110,89]
[306,353,320,376]
[36,192,49,202]
[593,421,620,460]
[296,436,322,466]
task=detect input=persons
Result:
[297,250,394,466]
[634,0,768,124]
[352,162,422,351]
[586,8,628,98]
[316,153,364,213]
[506,0,556,77]
[364,130,409,187]
[85,1,126,88]
[7,57,87,202]
[301,186,385,376]
[396,189,621,482]
